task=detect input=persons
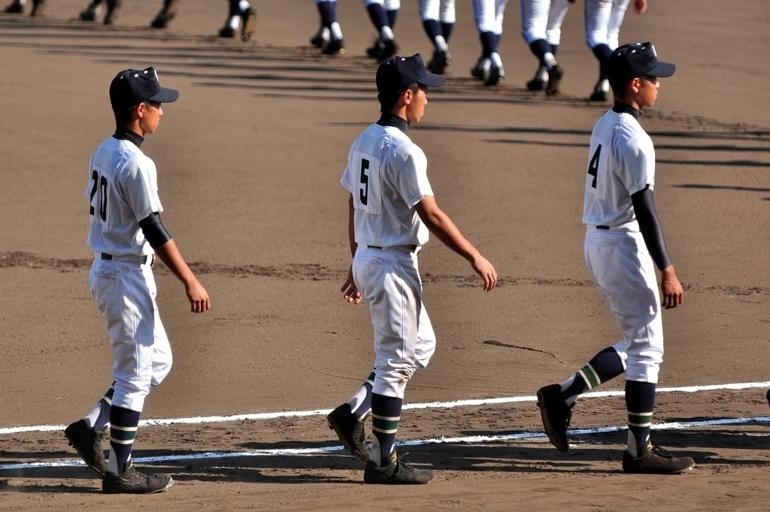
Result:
[4,0,48,18]
[519,0,571,96]
[325,52,501,487]
[362,0,402,64]
[469,0,510,88]
[79,0,121,26]
[417,0,457,75]
[568,0,649,102]
[218,1,258,43]
[63,65,209,495]
[309,0,345,56]
[150,1,178,29]
[535,37,696,475]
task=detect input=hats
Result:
[113,66,181,105]
[605,40,676,81]
[375,52,445,100]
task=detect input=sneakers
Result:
[594,87,610,102]
[363,460,436,485]
[425,52,455,73]
[623,444,696,474]
[366,35,399,64]
[218,7,257,42]
[526,64,563,96]
[63,419,109,475]
[0,0,186,30]
[470,63,505,87]
[536,383,572,452]
[310,34,345,56]
[101,468,175,494]
[327,403,373,459]
